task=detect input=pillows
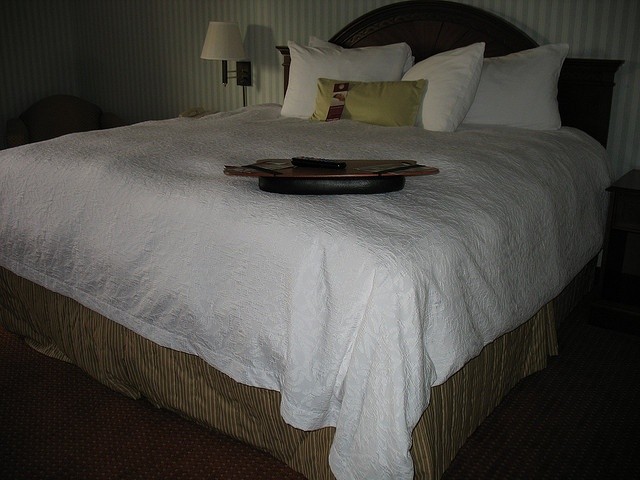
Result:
[400,41,486,133]
[307,35,345,48]
[311,78,427,128]
[461,44,570,130]
[281,41,413,118]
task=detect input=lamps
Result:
[199,21,252,87]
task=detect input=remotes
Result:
[291,155,347,170]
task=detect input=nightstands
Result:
[604,168,640,306]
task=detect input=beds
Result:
[1,1,624,475]
